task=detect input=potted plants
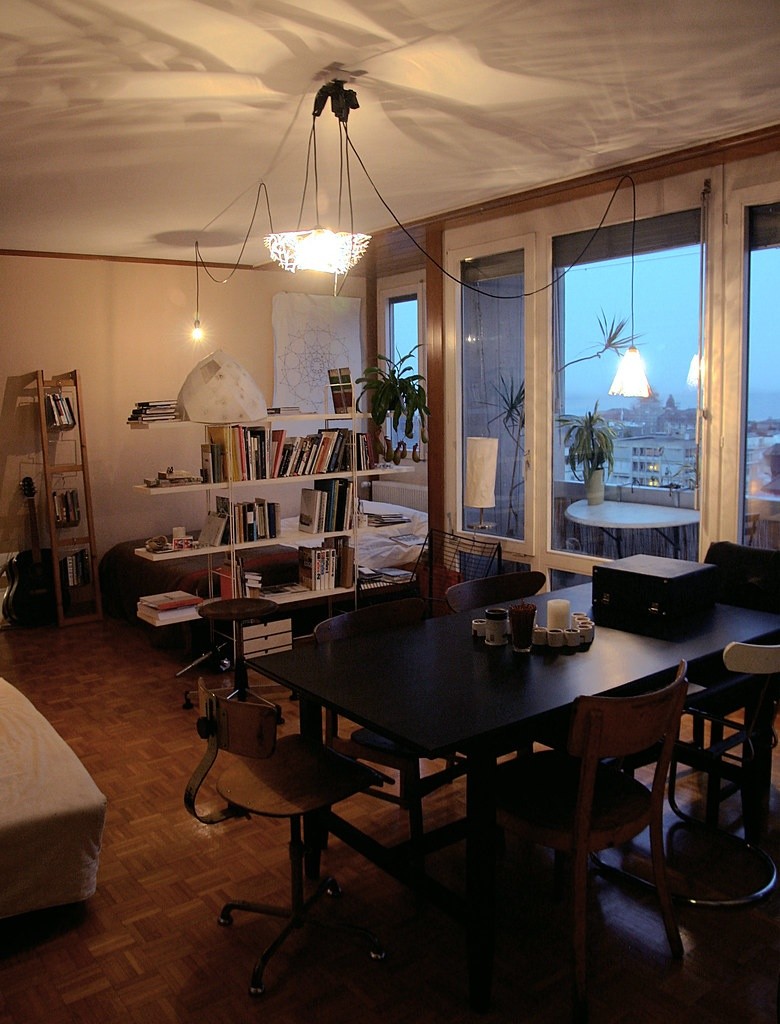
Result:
[354,343,432,443]
[554,400,620,506]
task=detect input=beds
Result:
[99,499,429,662]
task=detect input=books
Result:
[298,478,364,534]
[51,489,81,527]
[44,393,77,432]
[197,496,281,547]
[200,424,393,485]
[298,535,355,591]
[358,567,416,583]
[389,534,429,547]
[61,548,92,588]
[128,399,178,423]
[328,367,356,414]
[137,590,203,626]
[267,406,316,417]
[361,512,412,527]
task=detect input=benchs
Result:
[0,678,107,922]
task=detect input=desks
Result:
[564,498,701,560]
[243,579,780,1013]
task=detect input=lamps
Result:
[607,177,655,400]
[262,83,373,275]
[464,435,499,531]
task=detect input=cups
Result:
[484,608,508,645]
[577,625,592,643]
[575,617,590,628]
[578,621,595,638]
[509,603,537,652]
[532,627,547,645]
[471,619,485,637]
[547,599,571,630]
[571,612,587,628]
[547,629,564,647]
[173,527,186,538]
[564,628,580,646]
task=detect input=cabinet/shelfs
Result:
[127,410,417,658]
[32,366,106,629]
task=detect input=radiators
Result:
[370,478,428,513]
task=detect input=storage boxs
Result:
[591,554,721,630]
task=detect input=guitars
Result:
[1,476,71,629]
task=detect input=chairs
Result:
[495,658,691,1006]
[664,539,780,824]
[314,597,470,889]
[588,641,780,914]
[444,572,549,763]
[181,676,389,998]
[743,512,761,546]
